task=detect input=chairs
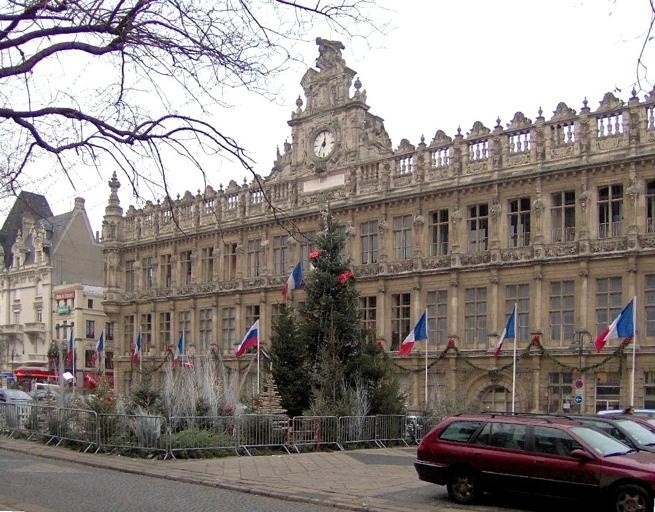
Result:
[551,440,570,456]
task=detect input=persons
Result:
[624,405,636,416]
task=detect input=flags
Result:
[595,298,635,352]
[95,331,104,360]
[132,331,142,364]
[170,332,184,369]
[65,332,73,365]
[496,309,515,358]
[279,260,302,298]
[400,312,428,357]
[234,319,259,357]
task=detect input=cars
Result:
[414,408,655,511]
[0,389,33,403]
[35,382,61,392]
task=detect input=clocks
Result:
[309,126,337,162]
